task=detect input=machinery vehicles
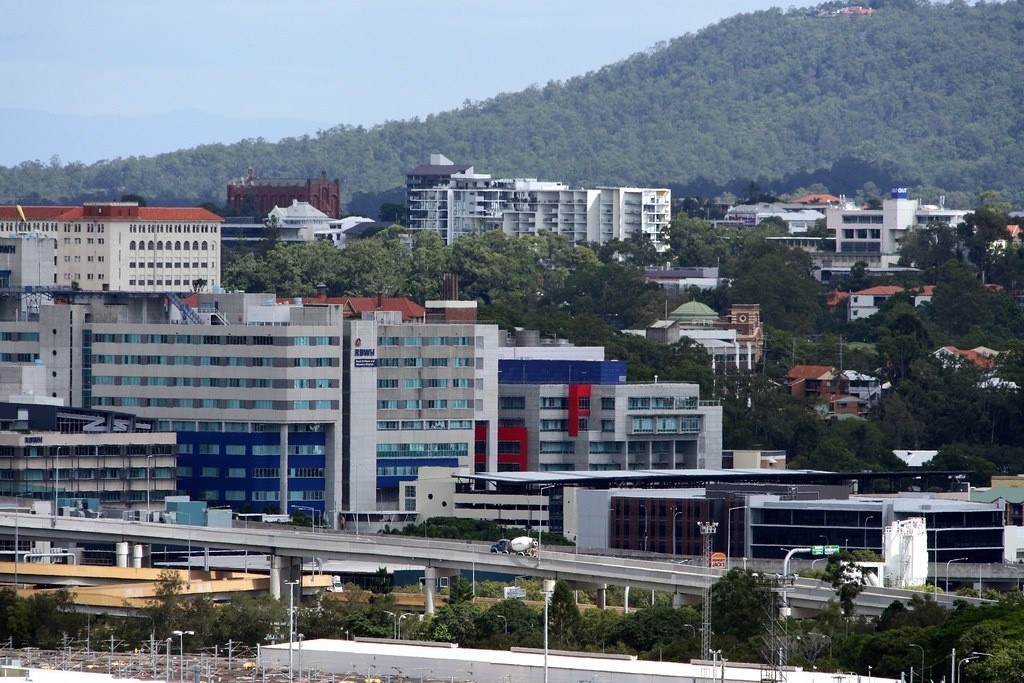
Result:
[491,536,539,559]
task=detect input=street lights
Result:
[864,516,874,551]
[946,558,968,594]
[290,504,315,532]
[172,630,195,683]
[497,614,507,633]
[147,454,153,522]
[167,510,191,589]
[298,633,304,683]
[820,535,829,545]
[673,511,683,560]
[539,486,555,551]
[4,515,18,595]
[399,612,411,639]
[383,610,397,640]
[780,548,791,574]
[979,562,991,597]
[55,446,61,517]
[1003,566,1019,589]
[728,506,748,570]
[907,643,924,683]
